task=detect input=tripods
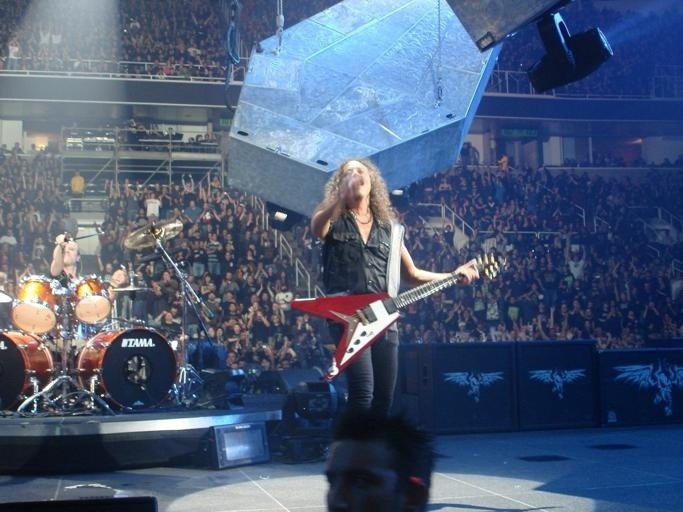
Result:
[153,236,214,409]
[17,281,115,416]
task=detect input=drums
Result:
[11,274,63,335]
[79,328,177,411]
[0,332,54,413]
[72,275,111,322]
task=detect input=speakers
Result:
[515,341,600,430]
[598,347,683,428]
[416,341,517,436]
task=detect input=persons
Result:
[322,414,436,512]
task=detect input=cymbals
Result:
[113,287,150,292]
[1,289,12,303]
[125,219,184,250]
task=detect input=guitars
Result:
[292,253,504,381]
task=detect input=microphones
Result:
[54,234,71,245]
